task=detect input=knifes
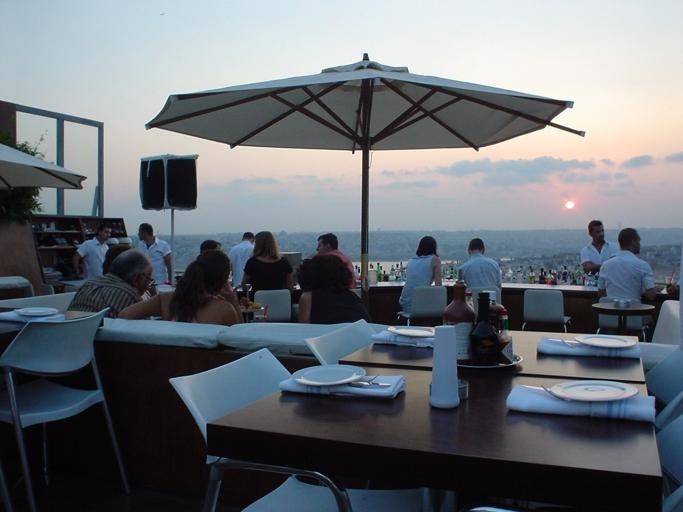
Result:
[351,380,391,386]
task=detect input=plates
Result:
[388,325,435,337]
[573,333,636,348]
[241,307,264,313]
[456,353,522,370]
[292,363,366,386]
[16,306,57,316]
[550,380,639,402]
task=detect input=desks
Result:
[592,301,654,342]
[238,305,264,322]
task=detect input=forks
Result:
[539,383,571,401]
[560,338,584,348]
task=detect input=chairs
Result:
[395,286,447,328]
[252,290,295,324]
[0,306,130,512]
[304,318,374,366]
[519,289,572,332]
[169,346,427,511]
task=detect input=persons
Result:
[457,238,503,289]
[580,219,658,325]
[66,223,373,326]
[398,235,443,313]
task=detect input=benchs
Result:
[0,287,367,504]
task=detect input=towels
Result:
[0,308,67,327]
[536,334,643,361]
[272,366,409,403]
[502,383,657,423]
[371,328,436,348]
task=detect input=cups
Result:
[41,224,46,232]
[49,223,55,232]
[429,326,461,407]
[664,275,672,291]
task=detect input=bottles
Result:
[503,265,598,287]
[353,261,457,288]
[442,279,512,364]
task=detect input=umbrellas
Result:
[0,139,86,193]
[143,49,587,295]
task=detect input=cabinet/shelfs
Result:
[30,212,85,285]
[80,215,127,242]
